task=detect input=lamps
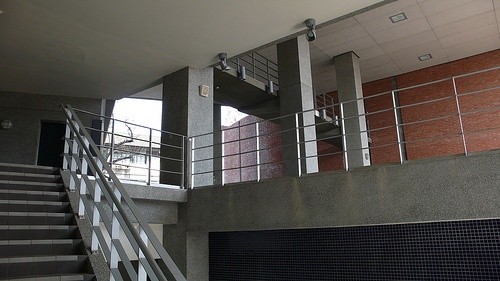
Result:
[265,81,274,94]
[304,18,317,42]
[216,52,231,71]
[237,66,247,81]
